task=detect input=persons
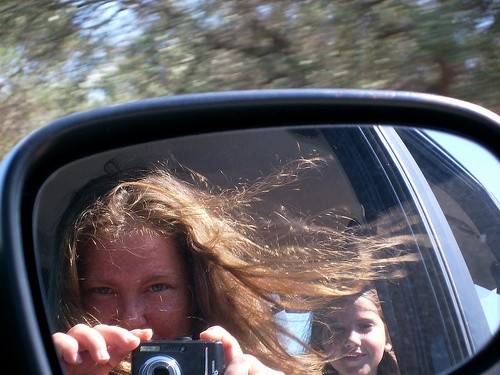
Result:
[50,163,399,375]
[310,286,400,374]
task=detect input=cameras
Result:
[131,337,226,374]
